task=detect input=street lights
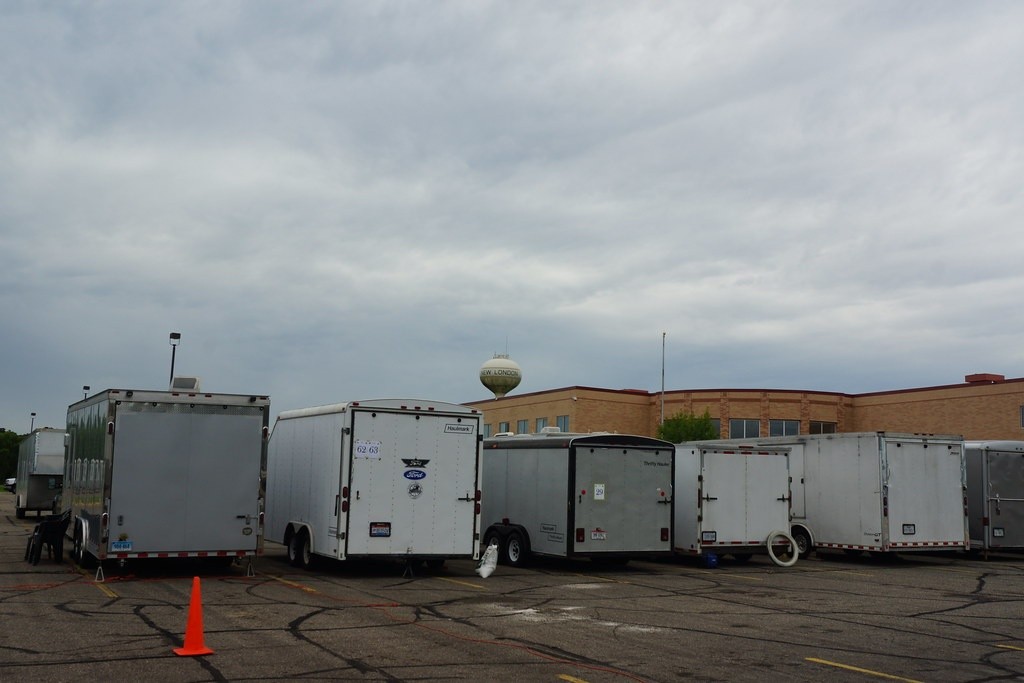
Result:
[83,385,90,399]
[31,411,36,433]
[168,332,181,386]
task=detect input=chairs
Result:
[25,508,73,567]
[81,508,100,545]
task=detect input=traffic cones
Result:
[171,576,214,657]
